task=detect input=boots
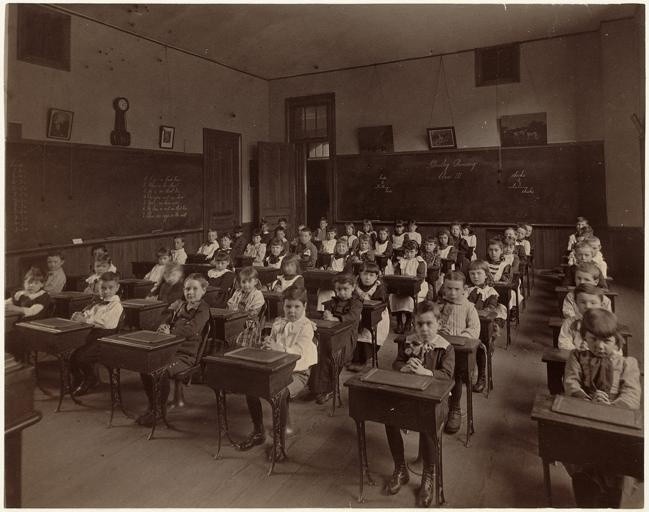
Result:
[382,458,410,496]
[417,463,436,508]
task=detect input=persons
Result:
[232,291,316,463]
[381,305,456,509]
[559,217,641,507]
[141,214,532,433]
[72,244,125,397]
[6,248,66,364]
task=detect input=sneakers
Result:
[314,392,334,405]
[269,442,286,461]
[396,321,404,334]
[404,321,412,333]
[472,378,487,392]
[347,362,366,372]
[65,371,98,395]
[135,408,166,426]
[445,408,462,433]
[237,432,266,450]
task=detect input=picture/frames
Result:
[46,107,74,142]
[427,126,457,149]
[159,126,175,149]
[475,41,520,86]
[16,4,71,72]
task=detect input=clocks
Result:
[111,97,131,147]
